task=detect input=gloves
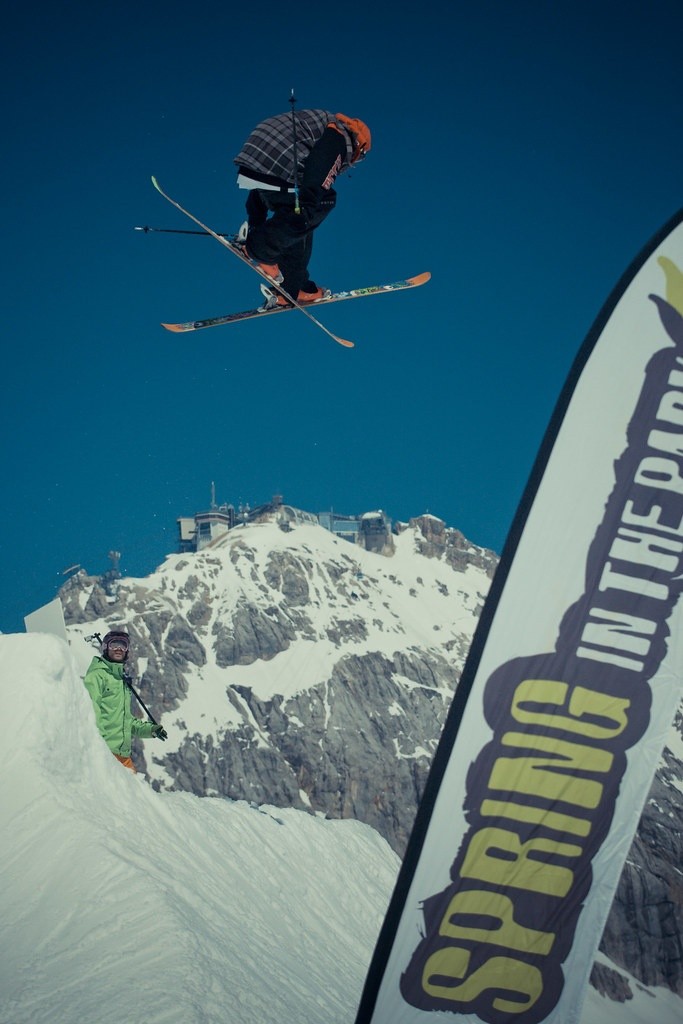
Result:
[299,194,320,216]
[152,724,168,741]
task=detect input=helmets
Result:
[102,631,129,663]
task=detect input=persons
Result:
[83,631,168,774]
[234,108,372,307]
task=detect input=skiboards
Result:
[147,171,433,350]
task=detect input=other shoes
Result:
[269,282,325,303]
[237,236,279,278]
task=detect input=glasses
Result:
[108,639,128,651]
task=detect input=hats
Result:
[346,116,371,165]
